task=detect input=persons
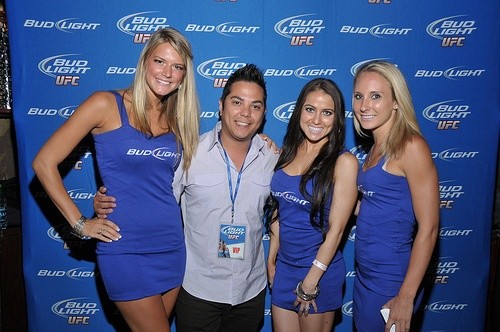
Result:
[93,62,281,331]
[266,76,358,331]
[31,26,281,332]
[351,61,440,331]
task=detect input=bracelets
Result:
[311,258,328,273]
[294,280,321,301]
[69,215,87,238]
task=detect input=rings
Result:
[264,136,269,140]
